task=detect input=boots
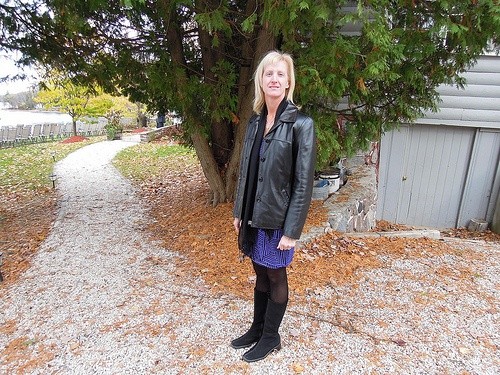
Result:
[231,287,270,349]
[242,297,289,361]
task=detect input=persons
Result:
[229,51,315,363]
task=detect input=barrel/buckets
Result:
[318,167,340,195]
